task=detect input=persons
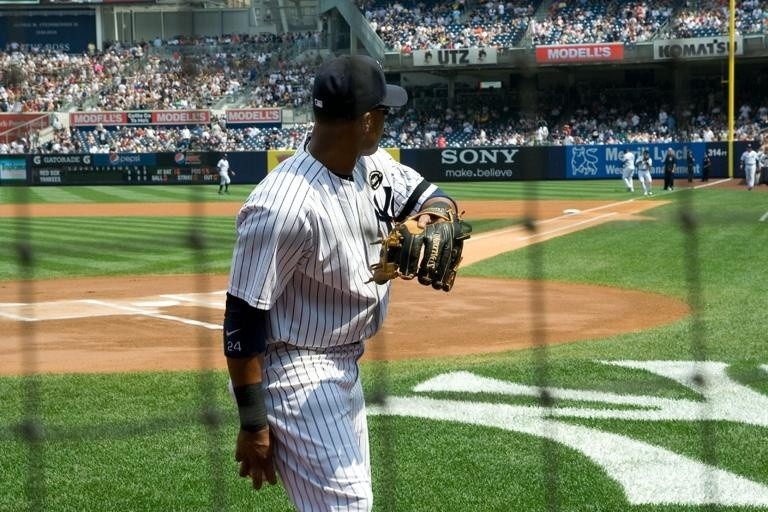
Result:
[356,1,768,52]
[702,154,711,181]
[636,151,652,195]
[0,31,320,155]
[217,154,235,194]
[664,149,676,190]
[387,69,768,148]
[740,143,768,190]
[687,152,696,182]
[622,151,635,192]
[224,55,474,512]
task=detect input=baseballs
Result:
[247,461,277,481]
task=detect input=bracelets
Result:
[232,382,267,433]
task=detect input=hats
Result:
[313,55,408,117]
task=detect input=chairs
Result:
[24,0,768,156]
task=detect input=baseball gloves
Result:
[230,171,236,176]
[367,201,472,291]
